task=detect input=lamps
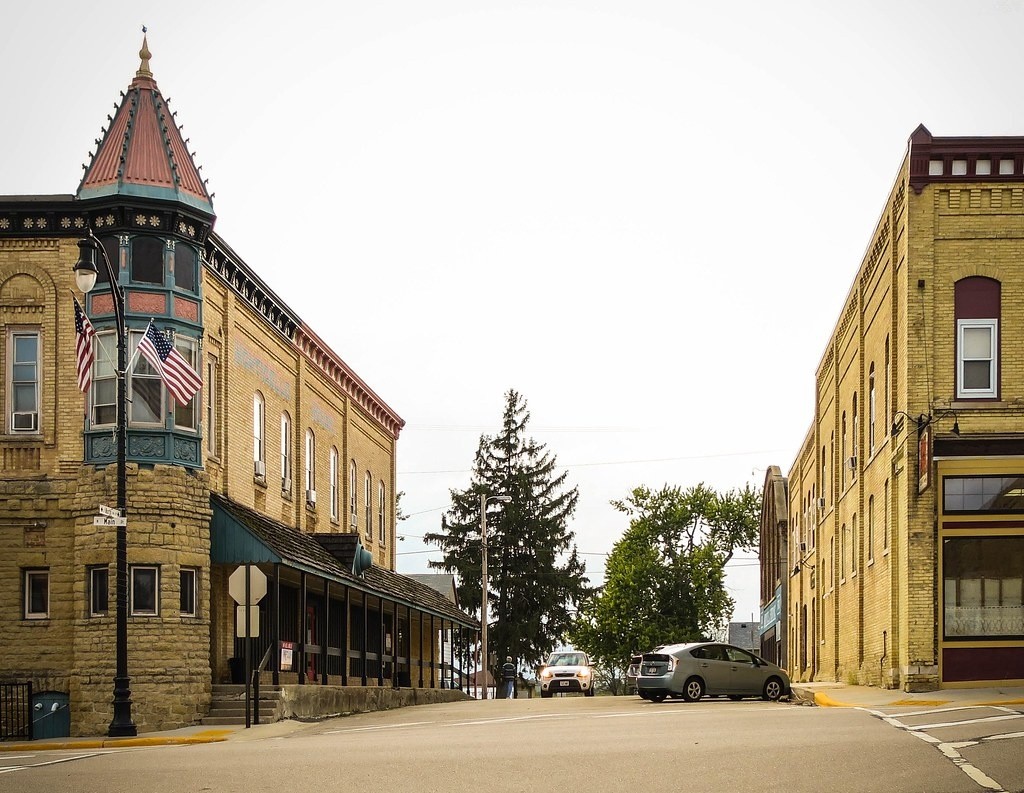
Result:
[889,407,963,439]
[789,558,815,578]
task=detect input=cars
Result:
[628,642,790,703]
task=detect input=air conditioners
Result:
[351,514,358,527]
[799,542,806,552]
[12,411,37,431]
[306,489,317,502]
[255,460,266,476]
[281,476,291,491]
[817,496,825,509]
[848,455,857,469]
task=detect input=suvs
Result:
[541,651,595,697]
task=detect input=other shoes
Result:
[506,697,510,699]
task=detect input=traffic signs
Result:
[92,517,127,527]
[100,504,121,518]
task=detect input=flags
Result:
[137,322,203,409]
[73,295,94,394]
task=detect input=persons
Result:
[500,656,517,699]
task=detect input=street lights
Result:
[71,222,137,737]
[481,493,512,699]
